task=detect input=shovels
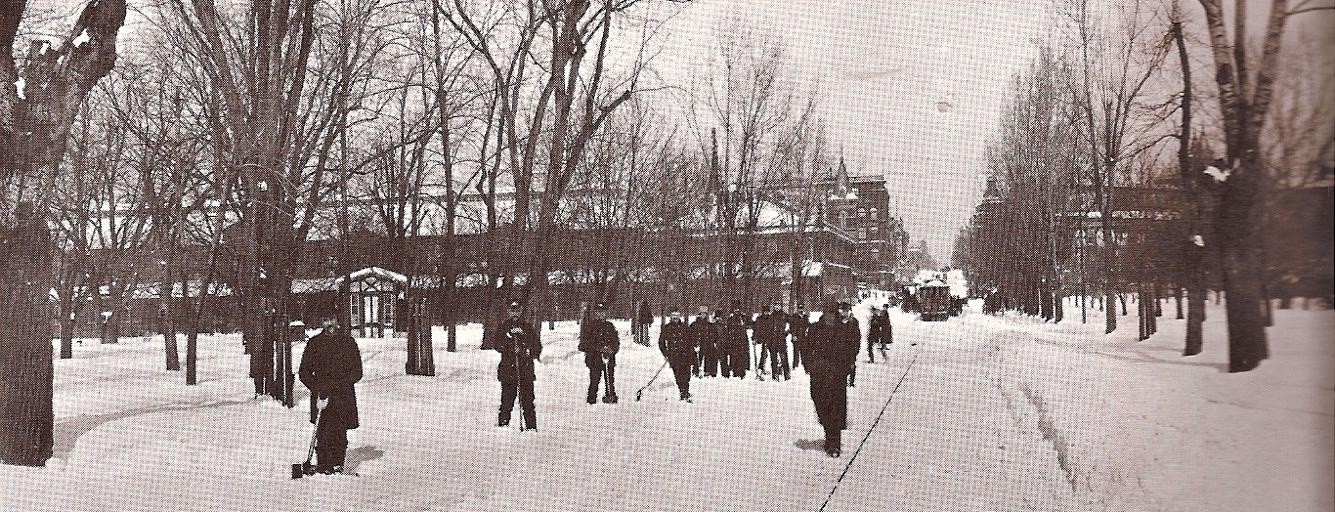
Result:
[292,392,330,480]
[752,339,764,381]
[637,353,672,402]
[602,353,618,404]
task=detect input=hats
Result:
[714,310,723,319]
[840,302,851,309]
[506,298,523,310]
[773,299,783,306]
[697,306,709,318]
[594,303,608,311]
[797,303,805,309]
[762,305,770,319]
[317,301,342,317]
[670,312,681,320]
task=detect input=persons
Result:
[949,295,964,317]
[490,297,543,435]
[295,302,362,477]
[638,300,813,400]
[799,300,857,461]
[889,287,921,314]
[839,303,892,386]
[577,301,620,406]
[982,292,1003,315]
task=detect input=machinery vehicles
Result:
[901,285,961,321]
